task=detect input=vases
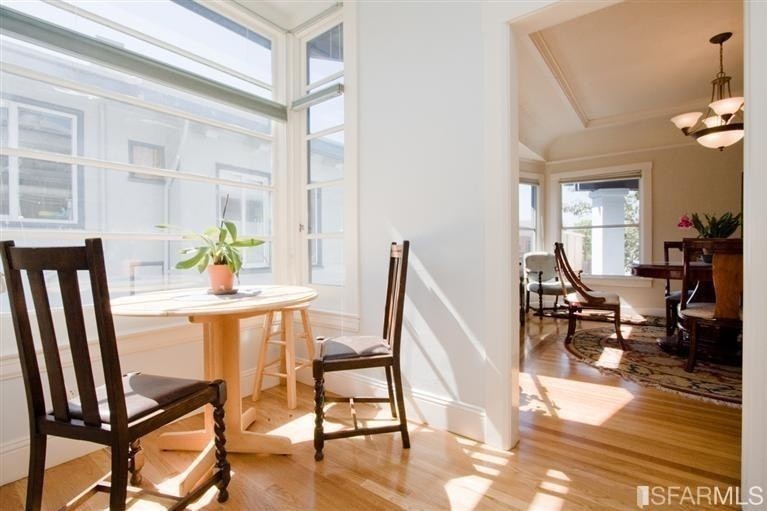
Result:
[703,247,714,264]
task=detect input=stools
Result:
[252,302,314,409]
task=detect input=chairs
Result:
[677,238,743,373]
[314,241,409,461]
[0,238,230,511]
[664,242,697,337]
[523,252,582,320]
[554,242,626,350]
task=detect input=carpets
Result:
[564,314,742,408]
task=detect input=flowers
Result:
[677,211,742,239]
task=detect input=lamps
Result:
[670,32,744,151]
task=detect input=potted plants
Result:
[153,193,266,295]
